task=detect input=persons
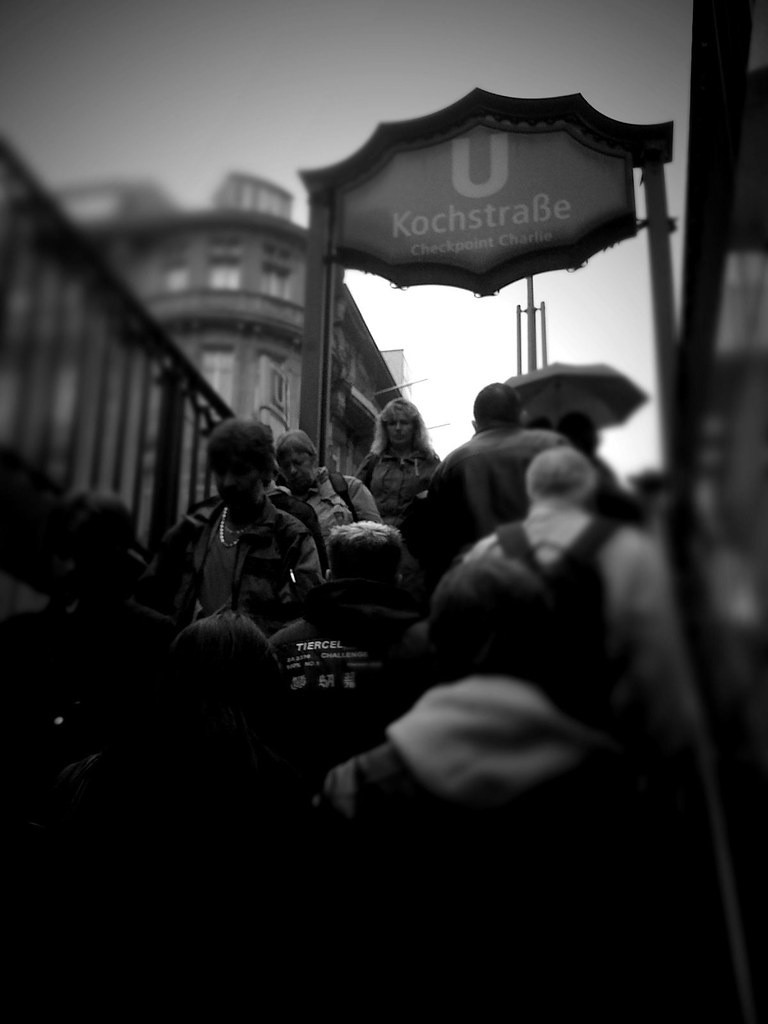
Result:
[0,386,728,1022]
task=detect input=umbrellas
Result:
[500,362,643,422]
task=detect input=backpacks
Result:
[497,519,607,657]
